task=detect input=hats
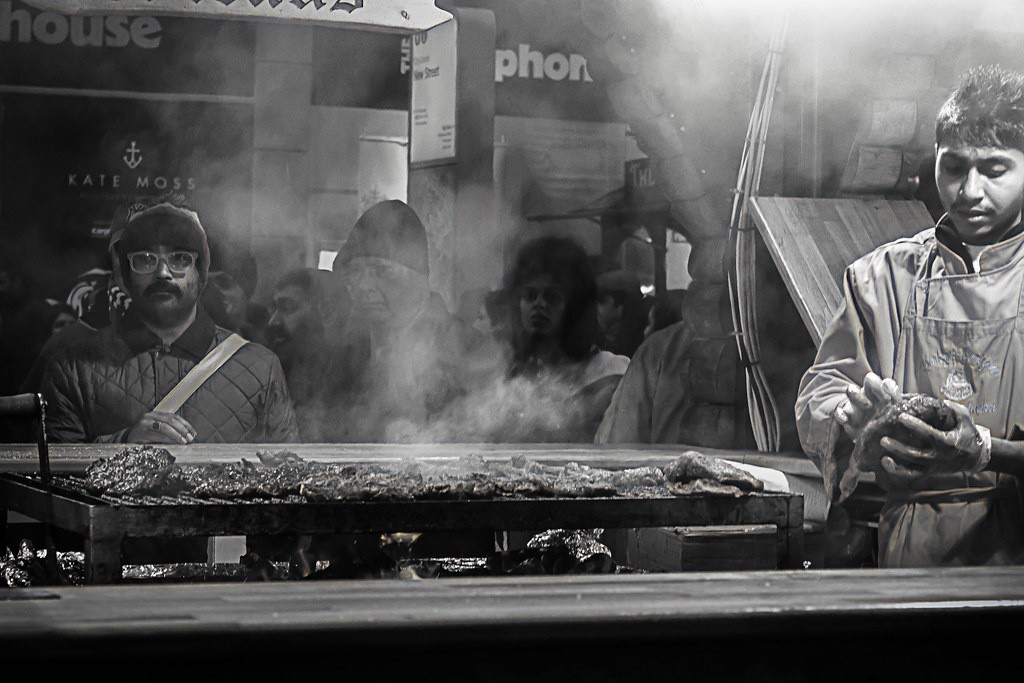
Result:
[347,199,430,276]
[105,196,210,297]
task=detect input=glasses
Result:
[126,250,198,274]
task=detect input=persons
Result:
[0,199,698,445]
[796,64,1024,570]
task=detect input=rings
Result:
[151,420,161,431]
